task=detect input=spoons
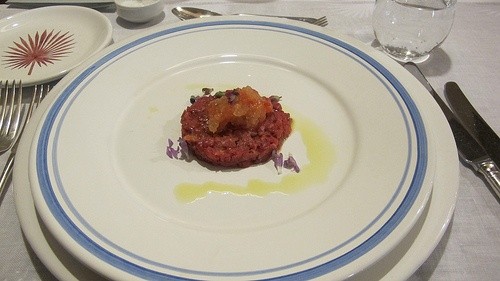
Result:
[172,7,316,23]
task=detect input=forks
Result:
[0,84,54,206]
[314,16,328,28]
[0,81,23,153]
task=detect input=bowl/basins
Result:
[115,0,163,23]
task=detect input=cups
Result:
[372,0,457,64]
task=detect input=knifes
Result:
[403,62,500,203]
[446,81,500,169]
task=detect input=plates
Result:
[28,16,434,281]
[5,0,115,8]
[0,5,113,89]
[12,24,461,281]
